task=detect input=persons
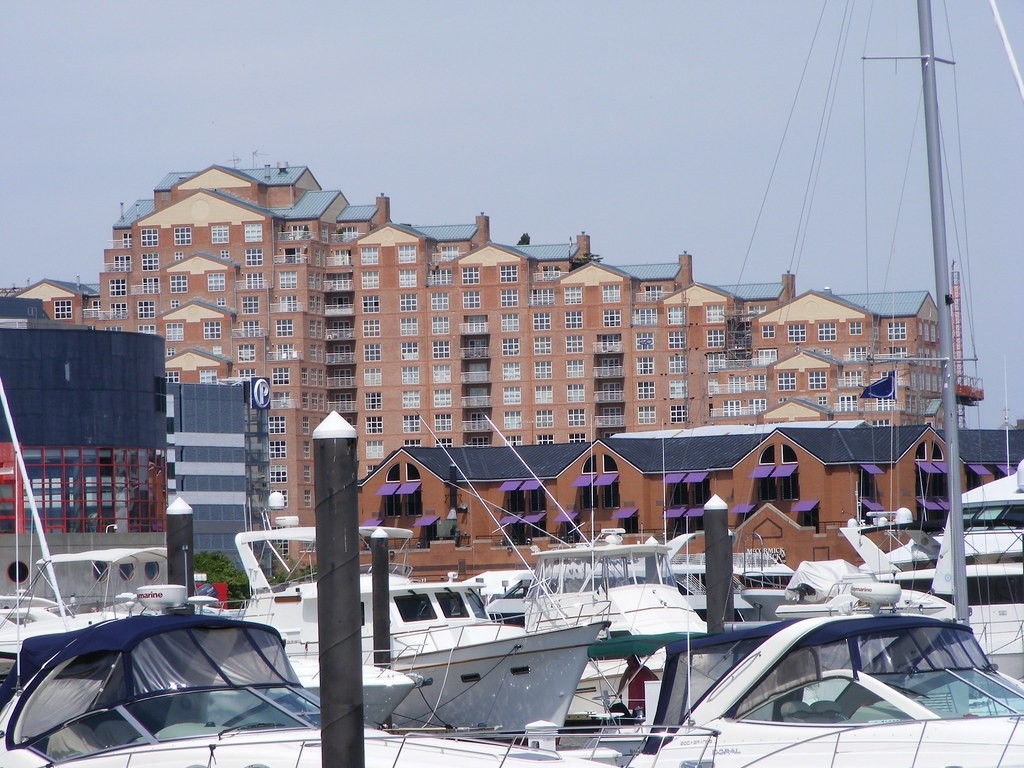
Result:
[616,657,660,717]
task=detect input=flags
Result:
[860,371,896,399]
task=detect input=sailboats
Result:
[0,0,1024,768]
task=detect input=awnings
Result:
[362,459,1022,529]
